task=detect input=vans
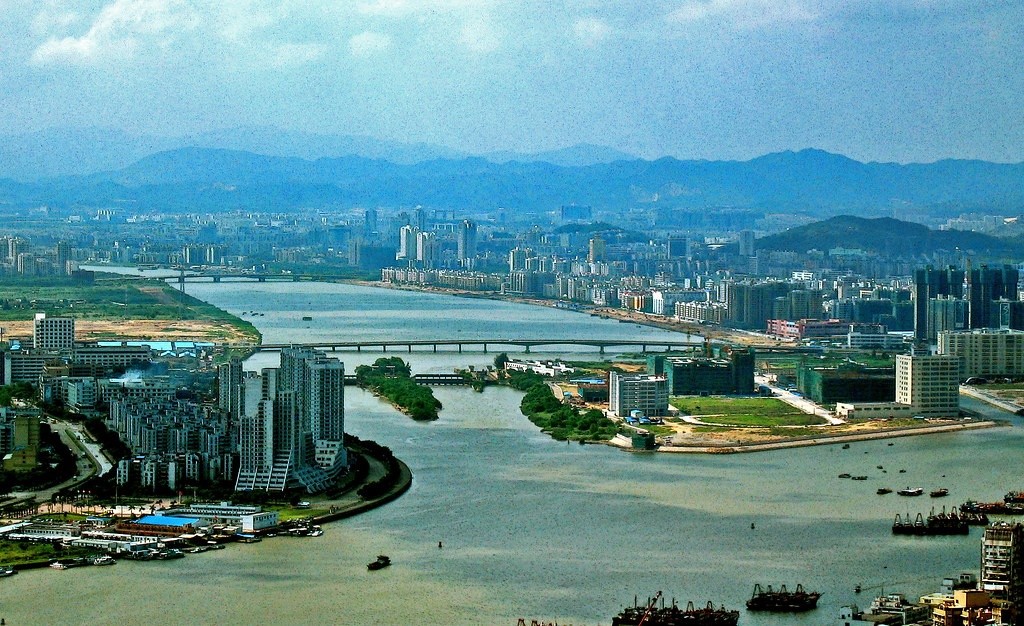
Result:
[965,377,978,385]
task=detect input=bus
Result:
[291,502,310,509]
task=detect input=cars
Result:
[978,378,987,384]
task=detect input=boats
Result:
[897,486,923,496]
[238,535,255,543]
[268,534,277,537]
[94,556,113,565]
[312,530,323,536]
[366,555,391,570]
[208,541,219,549]
[0,567,13,576]
[50,561,65,570]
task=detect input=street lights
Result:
[76,489,80,513]
[83,489,86,499]
[88,489,90,516]
